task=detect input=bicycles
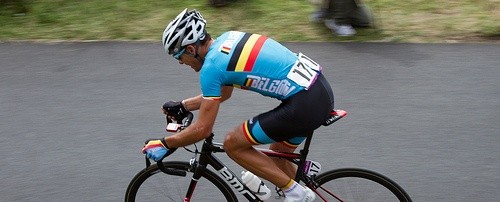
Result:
[124,108,414,202]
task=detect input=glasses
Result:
[175,44,197,61]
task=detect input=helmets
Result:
[162,8,207,56]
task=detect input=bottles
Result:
[241,171,272,201]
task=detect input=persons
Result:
[142,8,334,202]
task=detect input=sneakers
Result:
[283,186,316,202]
